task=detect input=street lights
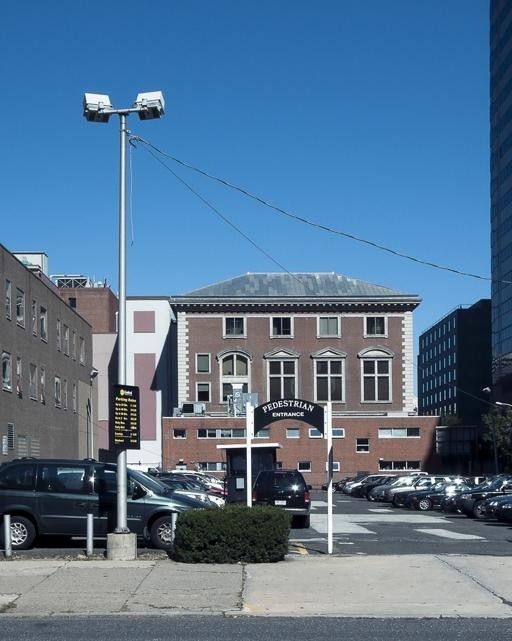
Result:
[83,87,168,560]
[494,401,512,407]
[482,386,500,475]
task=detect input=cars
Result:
[145,468,228,510]
[320,473,512,523]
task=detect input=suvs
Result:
[0,457,212,551]
[251,468,313,530]
